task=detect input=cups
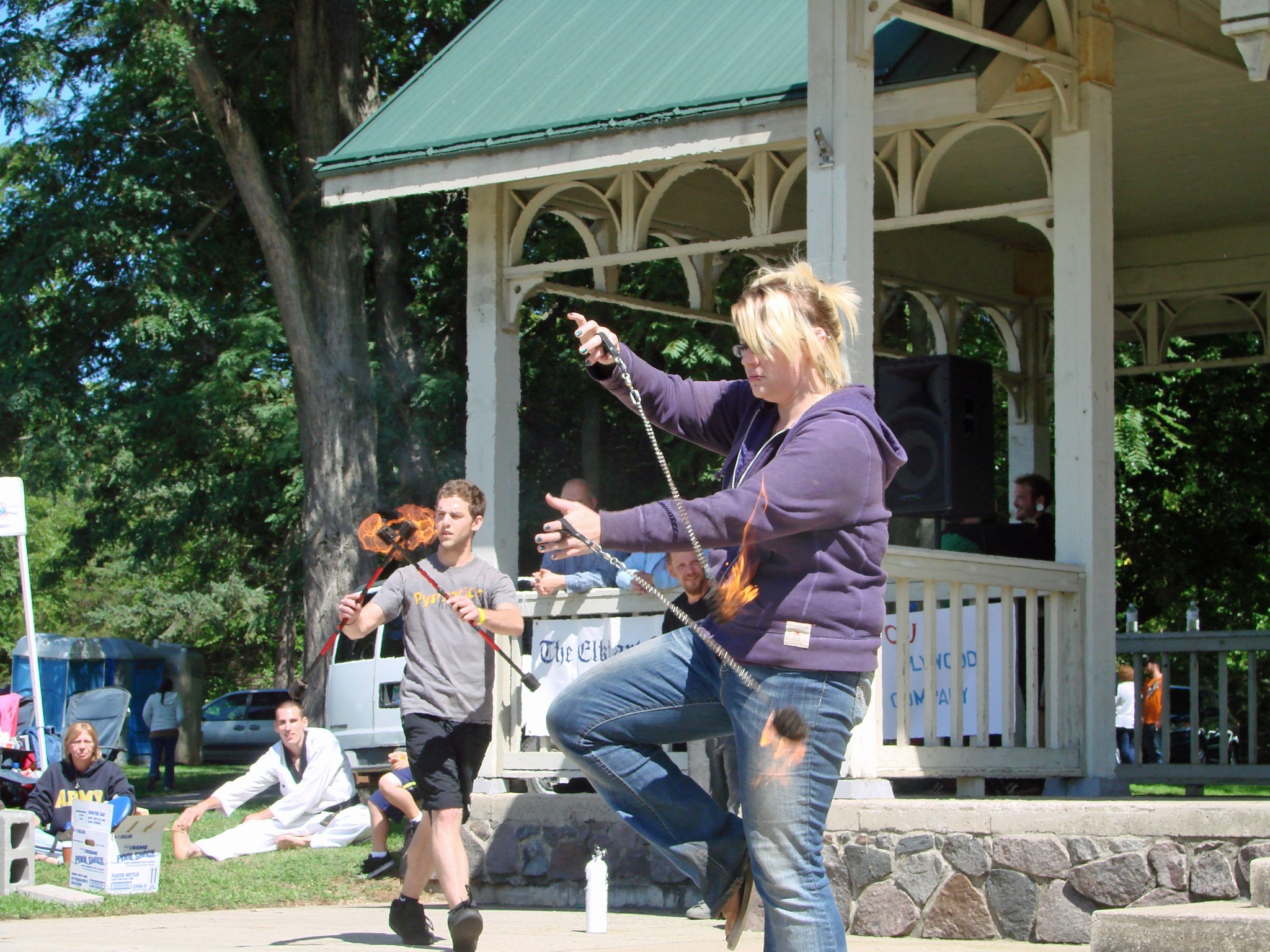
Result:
[62,840,72,865]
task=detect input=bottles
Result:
[1126,603,1138,634]
[585,843,608,933]
[1186,601,1200,632]
[11,762,21,773]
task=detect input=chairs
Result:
[0,687,133,808]
[17,696,34,732]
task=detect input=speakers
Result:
[872,353,997,520]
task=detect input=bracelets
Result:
[477,607,485,627]
[345,618,357,626]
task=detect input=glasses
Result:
[731,344,748,358]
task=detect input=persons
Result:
[959,471,1055,794]
[531,476,709,599]
[1142,657,1164,764]
[354,750,423,880]
[535,259,909,951]
[24,721,137,867]
[141,677,185,793]
[1114,664,1135,764]
[171,700,372,862]
[661,548,741,920]
[336,478,524,952]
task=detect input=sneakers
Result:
[401,821,419,858]
[388,899,435,945]
[355,850,396,879]
[447,885,483,952]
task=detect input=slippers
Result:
[727,856,754,951]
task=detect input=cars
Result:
[200,688,306,764]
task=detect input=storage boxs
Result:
[68,798,176,895]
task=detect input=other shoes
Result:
[685,900,712,919]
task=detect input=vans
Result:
[323,576,538,772]
